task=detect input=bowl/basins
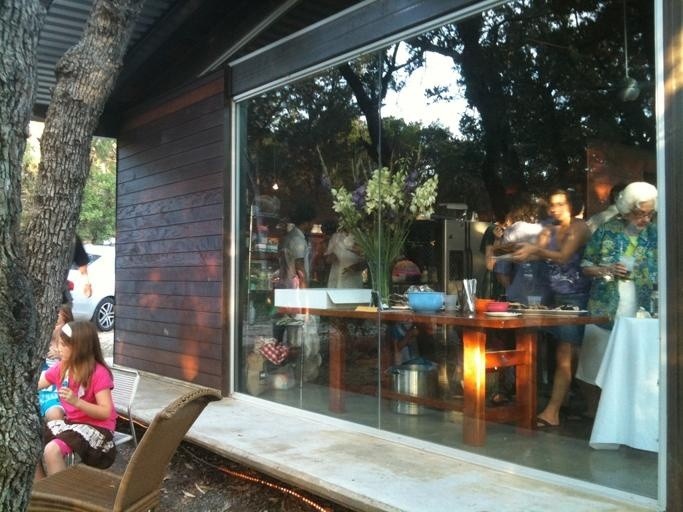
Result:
[474,299,494,314]
[487,302,509,315]
[404,292,444,314]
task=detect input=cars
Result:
[67,243,116,332]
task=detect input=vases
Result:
[367,261,393,308]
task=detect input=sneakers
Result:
[486,393,508,406]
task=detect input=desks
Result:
[279,307,609,448]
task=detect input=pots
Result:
[385,358,441,416]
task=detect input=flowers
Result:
[315,137,439,307]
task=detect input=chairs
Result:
[29,388,223,512]
[67,365,141,466]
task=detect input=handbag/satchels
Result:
[244,339,289,397]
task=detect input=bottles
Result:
[650,283,659,318]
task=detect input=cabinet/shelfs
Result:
[240,204,288,323]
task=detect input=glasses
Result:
[629,207,655,219]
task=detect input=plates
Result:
[391,305,409,310]
[509,304,588,317]
[490,253,521,262]
[482,311,523,318]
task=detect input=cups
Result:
[444,294,457,311]
[618,255,635,277]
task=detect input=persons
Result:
[317,219,344,272]
[574,182,657,427]
[320,216,370,290]
[479,189,592,421]
[34,305,74,437]
[585,183,627,234]
[513,189,601,428]
[277,207,316,287]
[30,317,120,485]
[53,232,92,319]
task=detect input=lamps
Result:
[615,0,641,103]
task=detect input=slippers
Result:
[536,416,561,428]
